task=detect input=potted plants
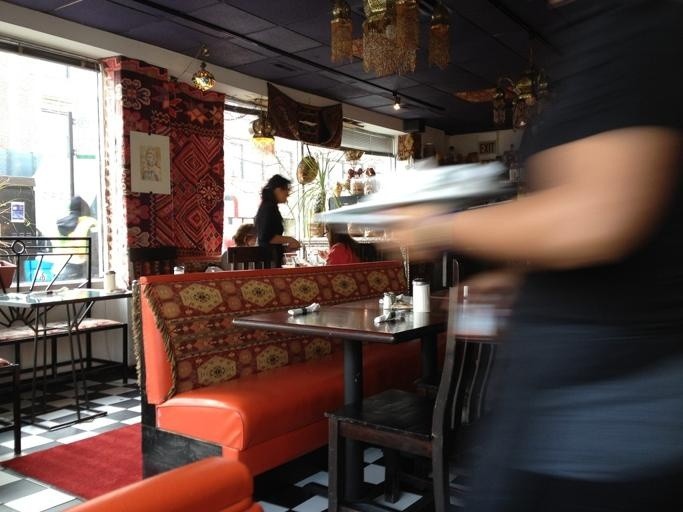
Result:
[0,178,17,289]
[285,143,346,238]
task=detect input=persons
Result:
[343,0,683,512]
[253,174,300,268]
[220,224,257,271]
[318,223,377,266]
[56,197,97,279]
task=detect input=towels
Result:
[374,311,406,325]
[7,293,28,301]
[288,302,320,315]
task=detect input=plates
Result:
[313,186,516,222]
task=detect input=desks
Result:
[0,288,133,429]
[232,289,467,504]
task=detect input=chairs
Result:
[228,246,265,270]
[130,247,177,280]
[323,256,495,512]
[0,356,22,455]
[413,365,477,491]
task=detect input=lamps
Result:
[191,42,216,92]
[329,0,453,77]
[248,100,277,146]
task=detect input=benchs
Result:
[0,237,128,389]
[131,260,449,498]
[63,456,265,512]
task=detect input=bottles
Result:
[411,276,432,313]
[173,266,185,275]
[104,270,115,291]
[383,291,396,311]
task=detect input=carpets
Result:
[0,423,143,501]
